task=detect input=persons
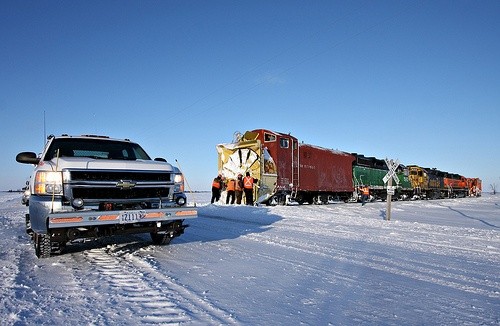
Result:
[242,172,258,205]
[234,174,243,204]
[211,174,224,204]
[226,179,235,204]
[358,185,369,206]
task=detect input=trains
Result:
[216,128,482,206]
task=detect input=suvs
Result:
[15,133,198,259]
